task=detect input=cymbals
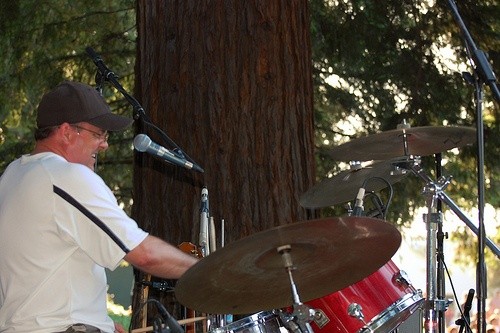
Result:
[298,160,408,209]
[175,216,403,315]
[328,125,478,162]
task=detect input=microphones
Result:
[133,134,204,173]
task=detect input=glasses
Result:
[72,125,109,142]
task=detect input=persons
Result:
[419,292,500,333]
[0,81,200,333]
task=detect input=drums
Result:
[208,309,281,333]
[281,258,426,333]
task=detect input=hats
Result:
[37,80,134,131]
